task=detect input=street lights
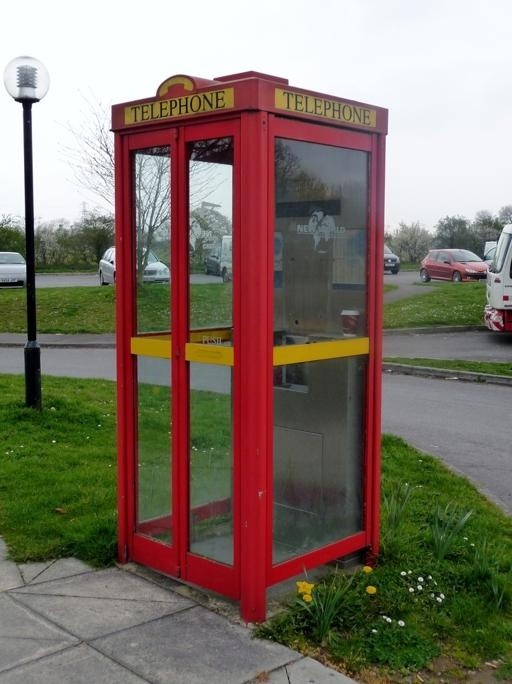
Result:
[4,56,50,408]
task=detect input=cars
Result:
[384,245,400,274]
[419,249,487,282]
[206,235,232,282]
[1,251,28,289]
[98,246,169,285]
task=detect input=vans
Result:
[484,225,511,331]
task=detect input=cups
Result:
[337,309,363,337]
